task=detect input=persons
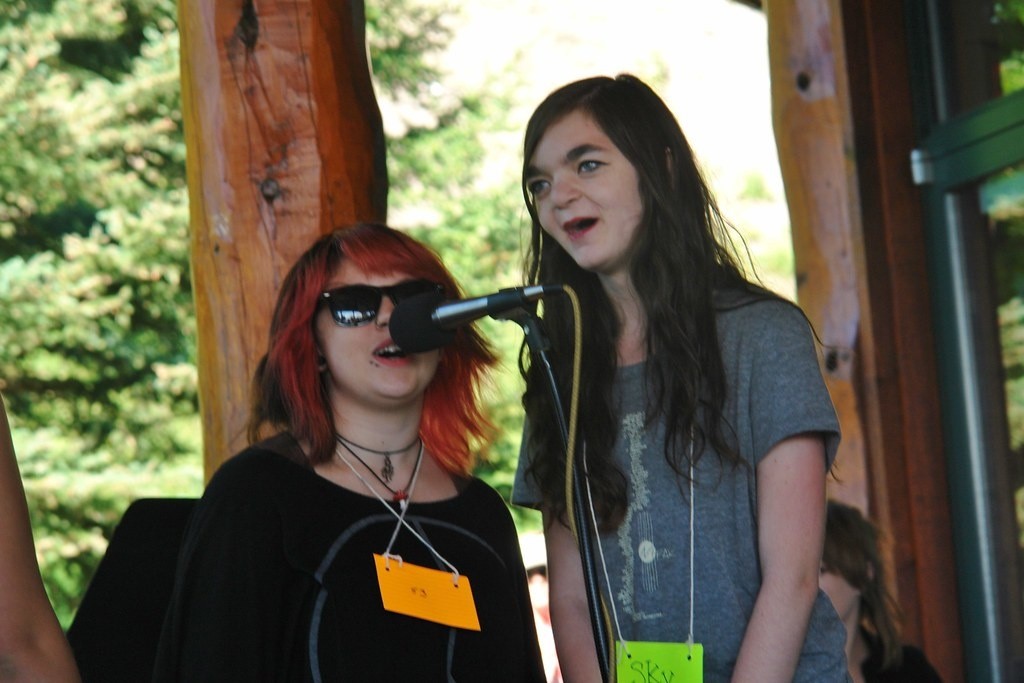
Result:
[511,73,854,683]
[149,223,549,683]
[819,499,950,683]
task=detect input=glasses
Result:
[317,278,444,327]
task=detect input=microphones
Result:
[388,283,563,356]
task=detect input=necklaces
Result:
[336,437,422,512]
[336,433,419,483]
